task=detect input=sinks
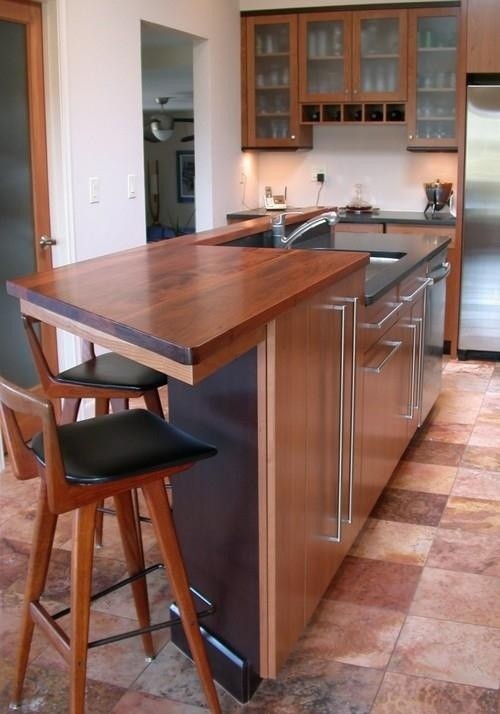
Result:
[311,245,407,284]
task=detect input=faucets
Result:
[270,211,339,249]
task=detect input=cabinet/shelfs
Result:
[239,3,468,153]
[458,3,500,360]
[227,203,459,355]
[6,214,455,703]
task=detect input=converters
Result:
[317,173,324,181]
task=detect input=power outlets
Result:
[310,165,326,183]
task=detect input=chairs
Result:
[21,311,172,553]
[2,377,220,713]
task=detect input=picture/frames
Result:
[174,148,199,205]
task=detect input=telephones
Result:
[263,185,287,209]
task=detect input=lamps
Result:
[244,157,254,177]
